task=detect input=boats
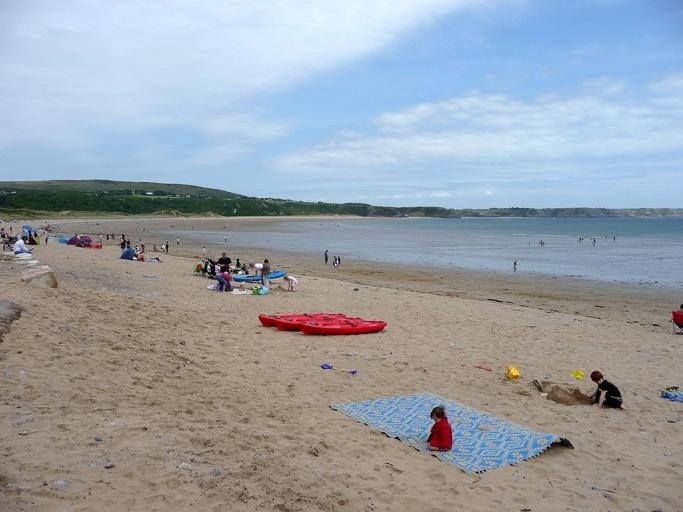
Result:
[232,270,287,282]
[258,313,386,335]
[88,242,102,248]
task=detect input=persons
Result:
[325,250,340,268]
[0,222,181,263]
[224,235,228,242]
[529,242,530,246]
[427,406,453,451]
[284,275,297,291]
[194,246,272,293]
[590,371,627,410]
[513,257,517,271]
[578,235,615,245]
[675,304,683,328]
[539,239,544,246]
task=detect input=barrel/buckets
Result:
[505,366,520,380]
[571,368,586,381]
